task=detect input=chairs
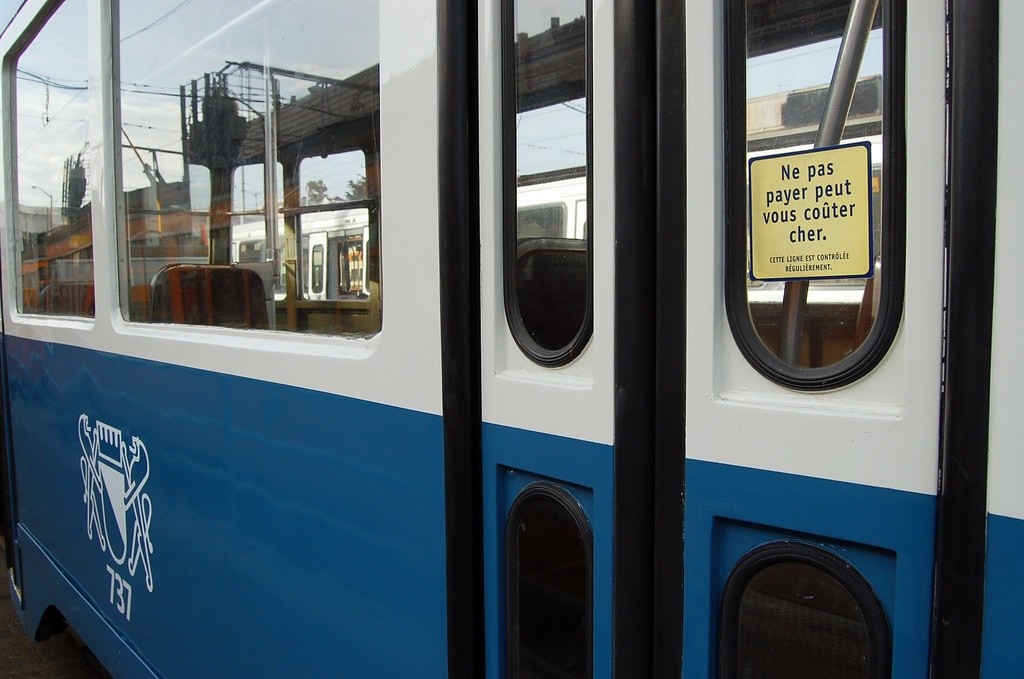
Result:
[515,233,589,347]
[39,281,94,318]
[146,261,270,329]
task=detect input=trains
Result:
[230,121,883,325]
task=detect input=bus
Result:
[0,0,1024,679]
